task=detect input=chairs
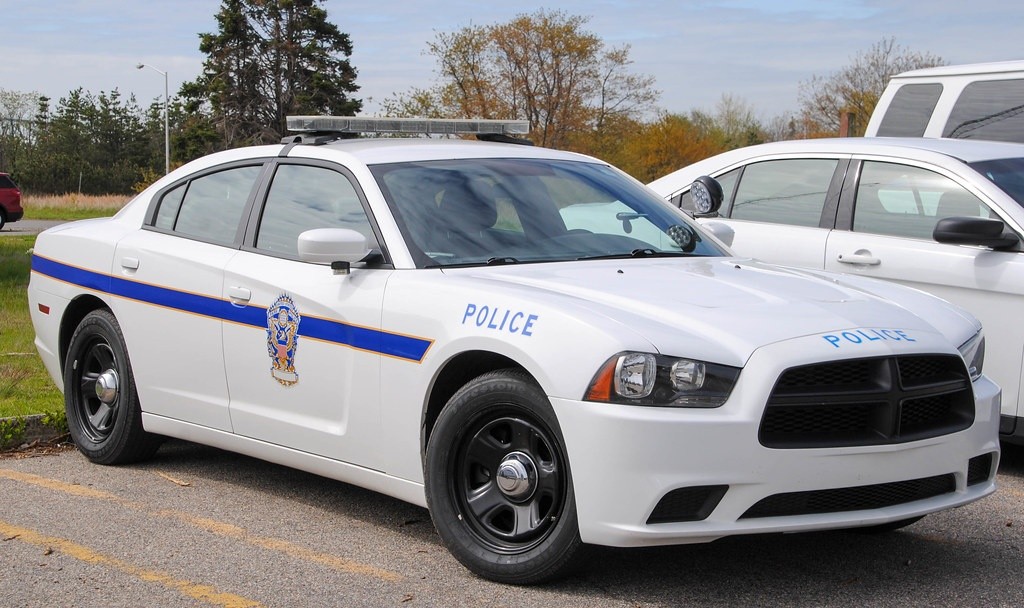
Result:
[429,178,504,260]
[936,188,981,219]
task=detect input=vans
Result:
[862,62,1024,224]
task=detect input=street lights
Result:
[137,61,170,178]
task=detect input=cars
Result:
[0,172,24,231]
[558,136,1023,450]
[26,117,1004,589]
